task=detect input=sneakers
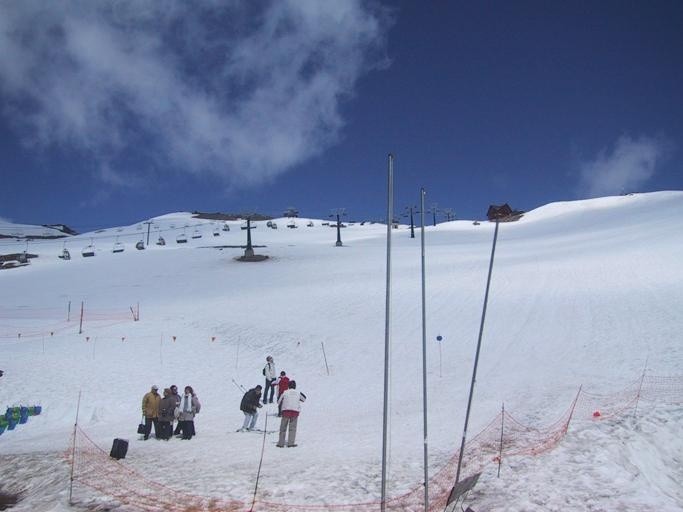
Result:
[276,445,284,448]
[288,444,297,447]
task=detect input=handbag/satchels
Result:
[262,363,269,375]
[110,438,127,460]
[137,414,147,434]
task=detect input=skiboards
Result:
[228,428,265,432]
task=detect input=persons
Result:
[262,355,276,404]
[141,384,201,441]
[240,385,263,432]
[274,380,307,448]
[270,371,290,417]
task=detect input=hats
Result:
[151,385,158,390]
[288,381,296,388]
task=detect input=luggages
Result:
[157,413,170,440]
